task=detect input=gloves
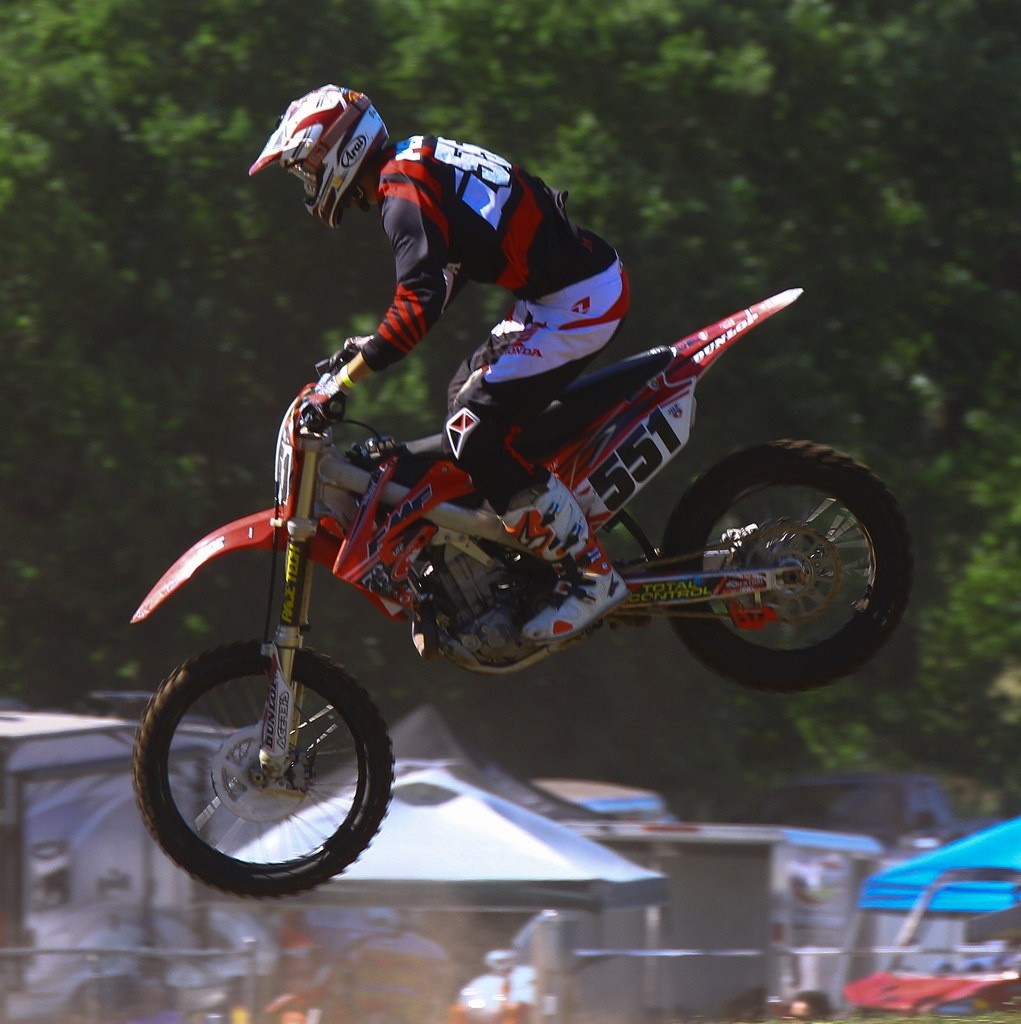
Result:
[297,374,346,438]
[343,336,375,356]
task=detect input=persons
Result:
[247,82,630,639]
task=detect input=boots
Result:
[496,474,630,645]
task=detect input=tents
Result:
[190,767,670,1024]
[1,709,296,994]
[826,819,1020,1013]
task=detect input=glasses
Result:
[288,161,323,201]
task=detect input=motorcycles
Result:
[127,283,919,898]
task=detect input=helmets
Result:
[248,84,387,228]
